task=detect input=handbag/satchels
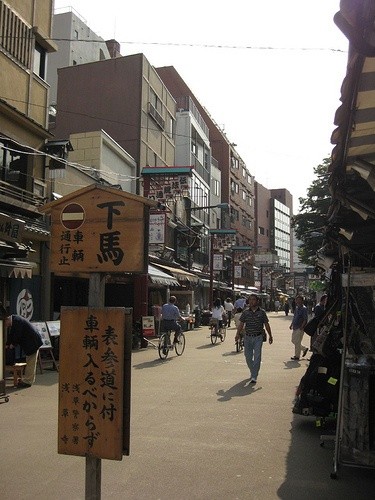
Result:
[292,289,375,416]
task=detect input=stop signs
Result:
[60,203,86,231]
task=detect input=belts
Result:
[246,332,262,337]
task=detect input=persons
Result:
[210,298,227,338]
[235,294,273,383]
[161,296,186,354]
[260,297,316,316]
[233,306,246,343]
[223,298,235,327]
[314,294,327,317]
[233,295,248,310]
[288,295,309,360]
[2,314,43,388]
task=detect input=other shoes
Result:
[302,348,308,357]
[250,379,257,383]
[173,338,181,343]
[162,349,168,356]
[18,382,31,388]
[290,356,299,360]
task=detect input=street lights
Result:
[191,203,229,210]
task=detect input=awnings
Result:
[148,262,259,293]
[326,0,375,217]
[1,259,36,280]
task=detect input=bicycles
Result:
[235,333,245,352]
[158,320,186,359]
[209,317,228,345]
